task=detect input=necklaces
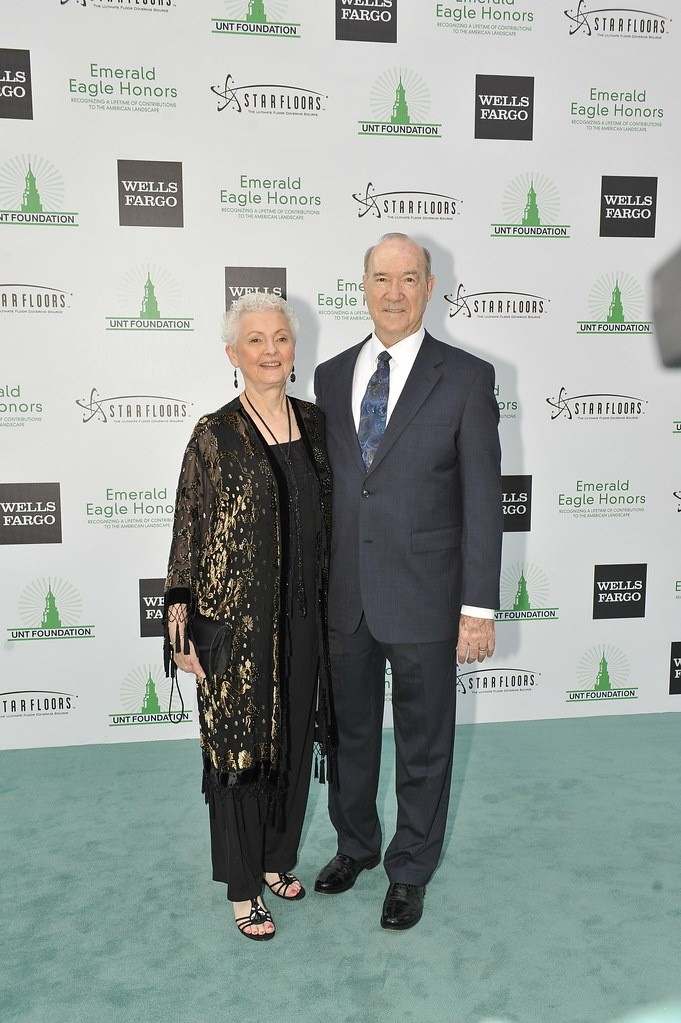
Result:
[244,390,308,619]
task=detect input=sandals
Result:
[235,897,275,942]
[262,872,306,901]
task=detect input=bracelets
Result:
[169,635,184,644]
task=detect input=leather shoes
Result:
[380,883,425,930]
[315,852,381,894]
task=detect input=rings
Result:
[478,647,487,651]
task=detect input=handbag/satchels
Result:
[188,615,232,676]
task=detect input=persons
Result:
[313,230,502,931]
[163,294,330,941]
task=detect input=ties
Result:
[358,351,390,474]
